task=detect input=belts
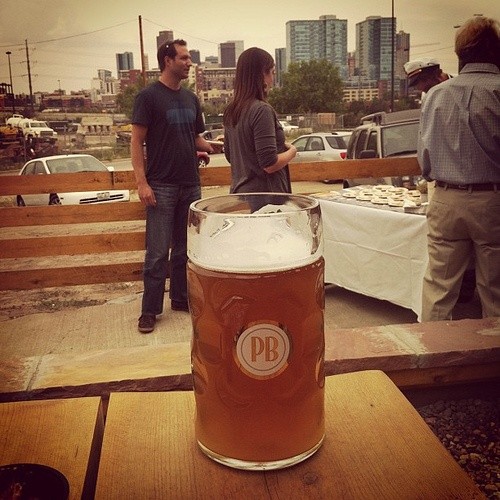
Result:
[435,180,500,192]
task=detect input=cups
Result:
[184,193,329,472]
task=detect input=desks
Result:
[320,186,431,321]
[92,367,487,500]
[0,395,104,499]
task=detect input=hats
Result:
[403,57,440,87]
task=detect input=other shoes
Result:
[170,299,188,312]
[139,313,156,332]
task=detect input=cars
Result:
[201,128,225,155]
[15,153,130,206]
[291,131,352,161]
[279,121,299,132]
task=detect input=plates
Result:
[342,184,422,207]
[0,462,70,500]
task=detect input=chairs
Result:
[69,161,80,171]
[56,165,67,173]
[311,141,321,150]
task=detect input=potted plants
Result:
[419,182,428,201]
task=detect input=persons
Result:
[417,16,500,323]
[403,58,456,95]
[131,39,213,332]
[222,47,297,213]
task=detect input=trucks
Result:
[346,108,421,159]
[6,116,59,145]
